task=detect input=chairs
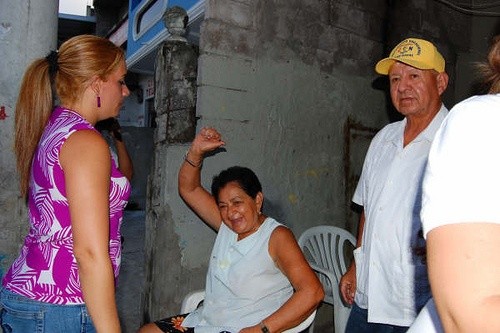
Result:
[180,287,317,333]
[297,225,358,333]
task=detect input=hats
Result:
[375,39,445,75]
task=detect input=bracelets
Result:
[183,151,202,168]
[258,320,270,333]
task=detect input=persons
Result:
[402,33,500,333]
[136,125,325,332]
[1,33,136,332]
[336,36,457,332]
[95,117,134,182]
